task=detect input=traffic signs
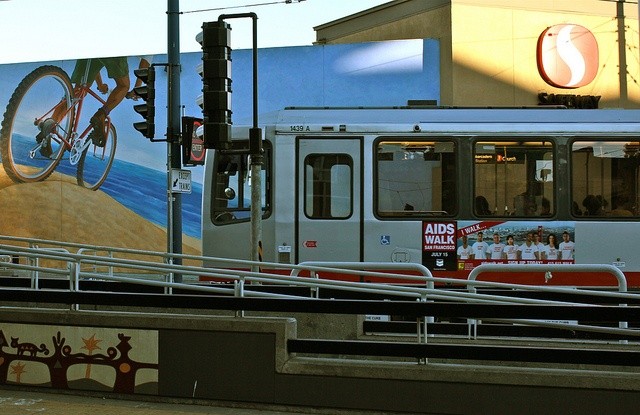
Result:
[168,168,193,195]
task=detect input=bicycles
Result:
[0,60,140,191]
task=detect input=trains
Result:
[196,98,640,290]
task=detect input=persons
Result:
[518,232,539,260]
[559,231,575,260]
[517,193,539,216]
[35,53,153,157]
[486,234,506,259]
[503,235,520,259]
[457,236,475,260]
[476,196,491,215]
[544,234,559,260]
[541,197,550,214]
[472,232,489,259]
[583,194,608,216]
[532,232,545,260]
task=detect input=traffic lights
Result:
[196,21,233,151]
[134,66,156,140]
